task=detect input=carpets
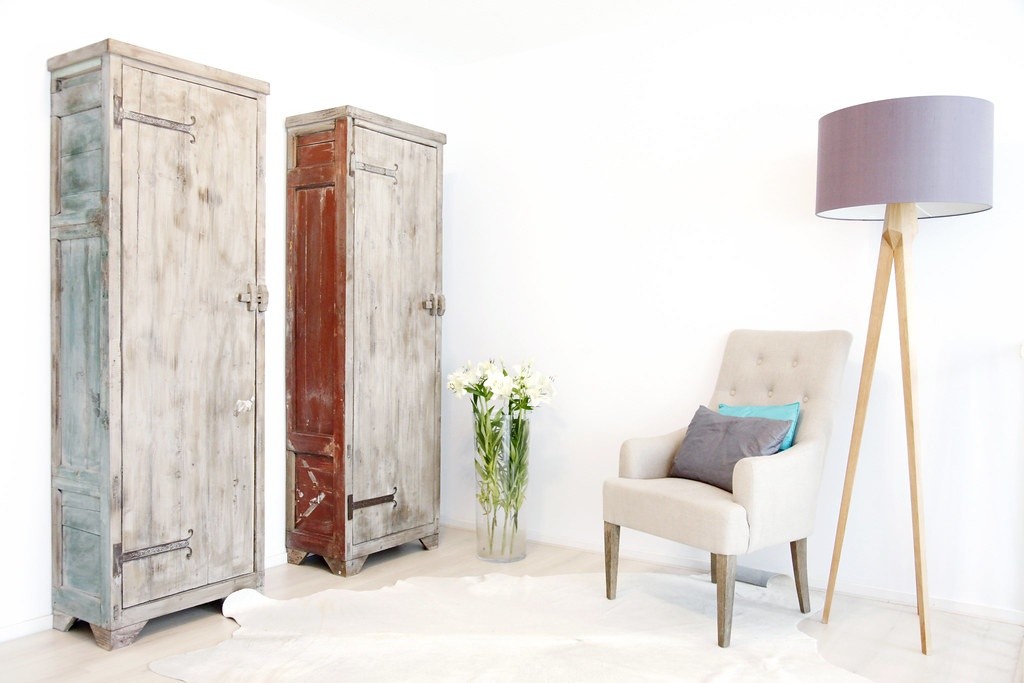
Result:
[147,565,872,683]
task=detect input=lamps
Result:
[815,95,995,656]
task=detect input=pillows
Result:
[668,405,793,495]
[718,401,801,452]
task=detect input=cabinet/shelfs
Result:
[47,39,270,650]
[286,106,446,577]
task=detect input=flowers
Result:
[447,359,556,558]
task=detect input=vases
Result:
[474,412,533,563]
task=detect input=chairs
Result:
[603,328,851,648]
